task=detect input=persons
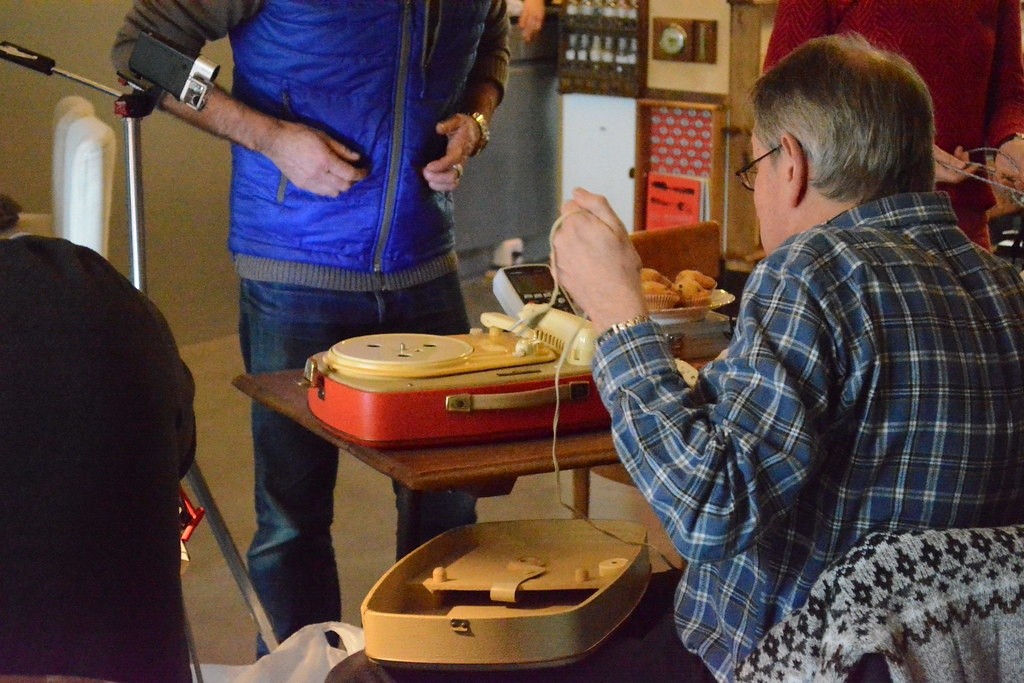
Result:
[111,0,512,664]
[762,0,1024,248]
[324,33,1024,683]
[0,233,195,683]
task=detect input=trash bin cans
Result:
[453,4,560,280]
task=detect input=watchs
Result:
[594,316,652,353]
[467,111,490,158]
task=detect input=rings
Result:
[452,164,462,179]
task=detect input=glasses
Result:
[736,141,803,190]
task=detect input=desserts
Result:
[639,268,717,310]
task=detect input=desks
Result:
[231,372,622,561]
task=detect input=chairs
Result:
[571,222,721,521]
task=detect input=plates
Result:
[648,288,737,326]
